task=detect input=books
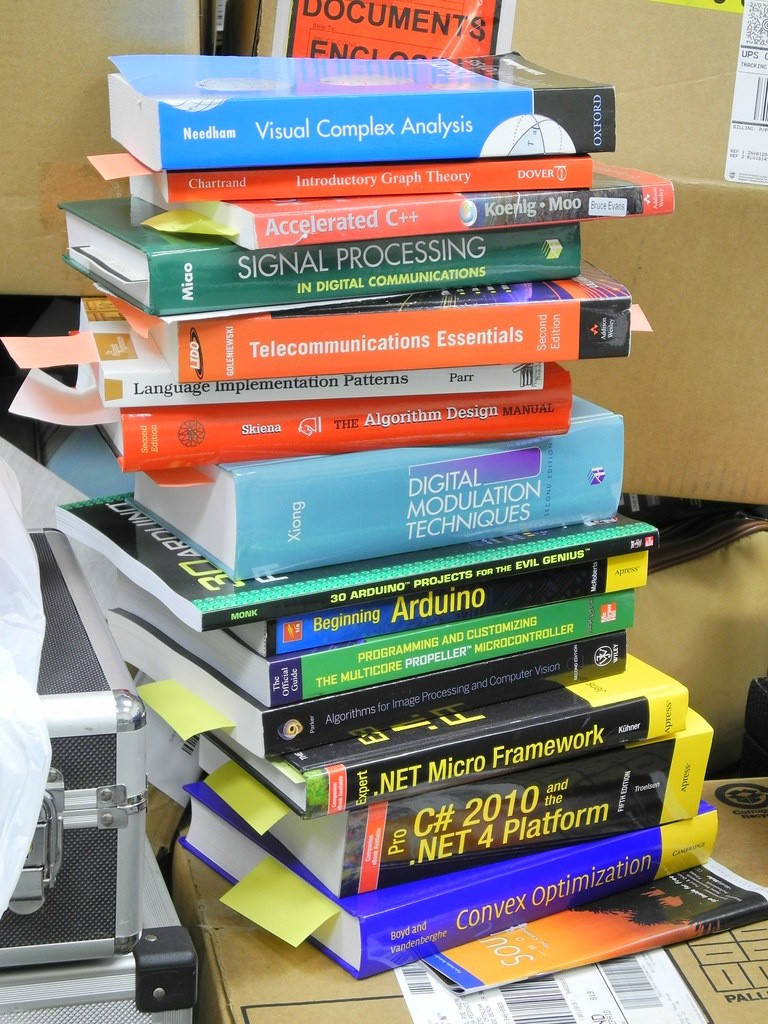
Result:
[179,781,719,980]
[418,857,768,997]
[129,161,674,251]
[203,652,689,819]
[107,53,616,172]
[124,395,625,583]
[150,257,632,383]
[102,551,653,760]
[160,154,593,204]
[78,296,545,409]
[58,196,581,317]
[199,708,715,899]
[103,362,571,473]
[56,492,660,633]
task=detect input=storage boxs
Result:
[171,776,767,1023]
[0,1,768,508]
[0,527,147,967]
[0,832,198,1024]
